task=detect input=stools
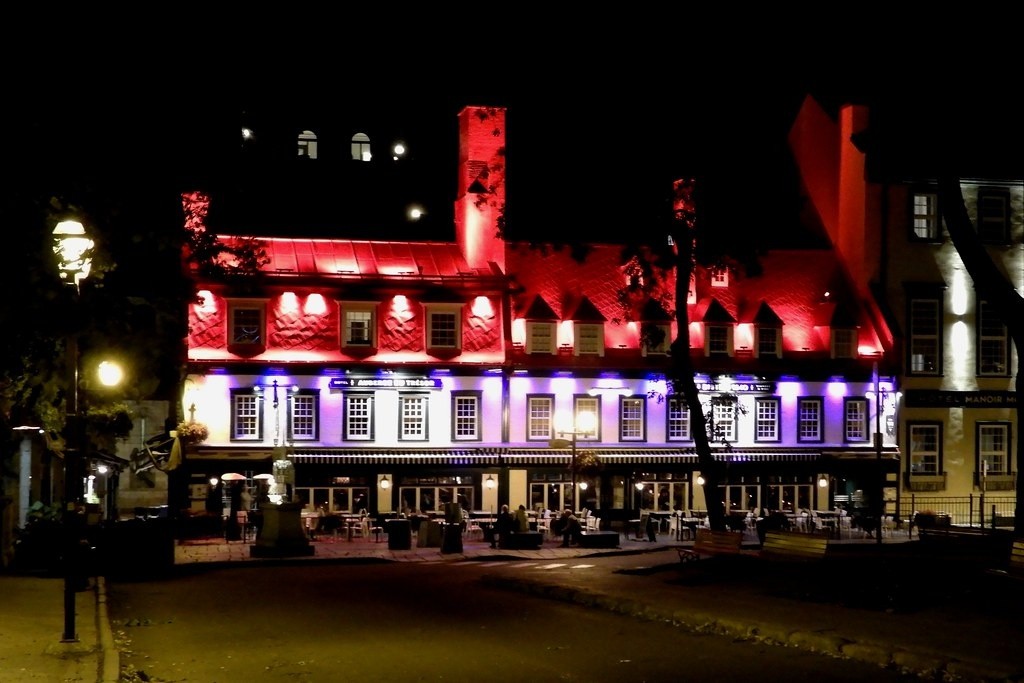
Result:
[369,526,382,543]
[506,531,542,547]
[579,531,619,548]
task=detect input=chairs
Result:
[676,514,691,541]
[575,509,601,532]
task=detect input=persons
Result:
[241,482,268,514]
[559,508,582,549]
[512,505,531,532]
[490,504,513,549]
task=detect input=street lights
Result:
[48,217,94,644]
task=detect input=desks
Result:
[649,509,899,539]
[302,509,562,541]
